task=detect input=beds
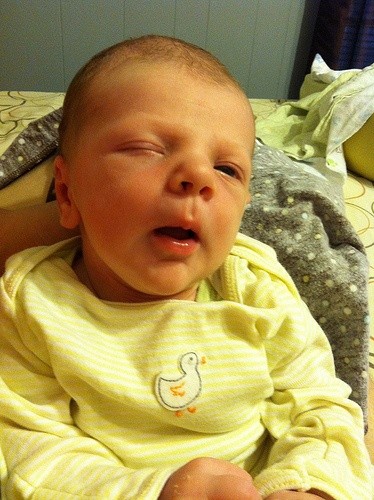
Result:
[0,54,374,500]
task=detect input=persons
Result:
[1,32,372,499]
